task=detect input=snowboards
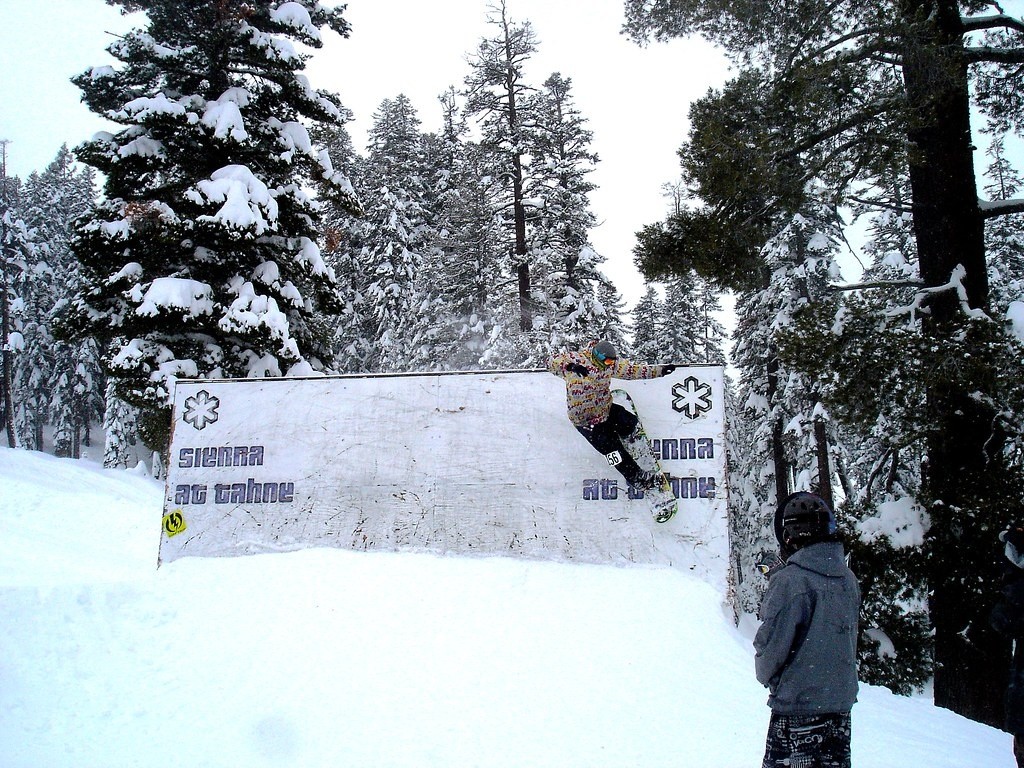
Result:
[610,389,678,523]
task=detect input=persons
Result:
[754,491,862,768]
[547,343,677,495]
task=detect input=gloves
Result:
[661,364,676,377]
[566,362,590,378]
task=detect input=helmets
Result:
[596,340,616,369]
[774,492,836,555]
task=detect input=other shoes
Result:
[632,470,665,492]
[623,423,641,445]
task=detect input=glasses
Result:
[757,564,770,575]
[603,357,618,368]
